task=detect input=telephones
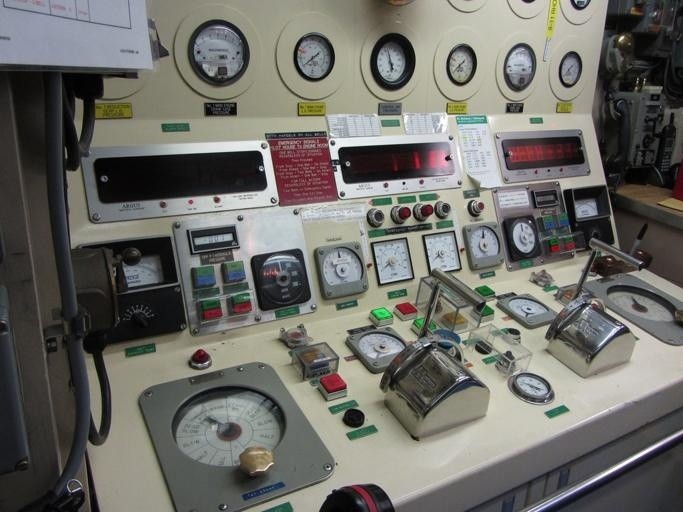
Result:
[649,113,676,187]
